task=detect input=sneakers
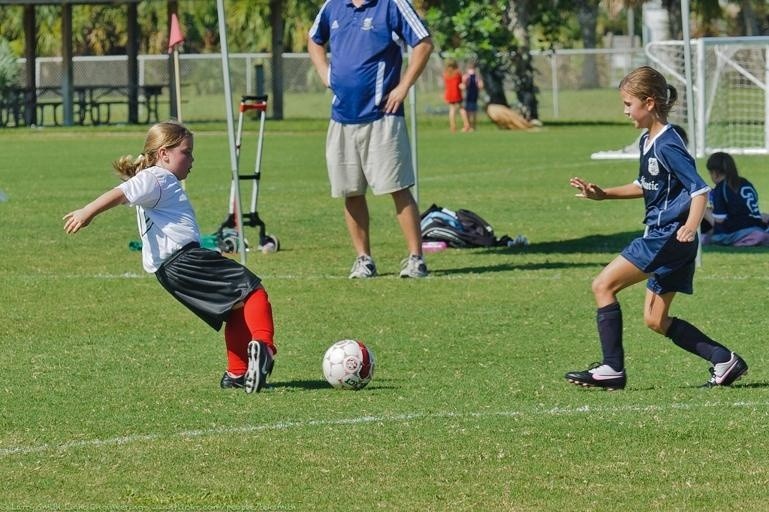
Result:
[566,362,627,390]
[221,370,245,389]
[400,252,428,277]
[349,254,379,278]
[244,339,274,396]
[704,352,748,388]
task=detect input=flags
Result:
[168,13,184,54]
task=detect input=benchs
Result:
[0,84,189,128]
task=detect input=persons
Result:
[61,121,278,395]
[443,57,464,134]
[458,53,485,133]
[565,65,748,392]
[701,152,769,247]
[307,0,435,279]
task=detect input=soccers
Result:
[322,339,375,391]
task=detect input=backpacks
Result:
[420,202,513,248]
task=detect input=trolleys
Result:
[208,96,280,256]
